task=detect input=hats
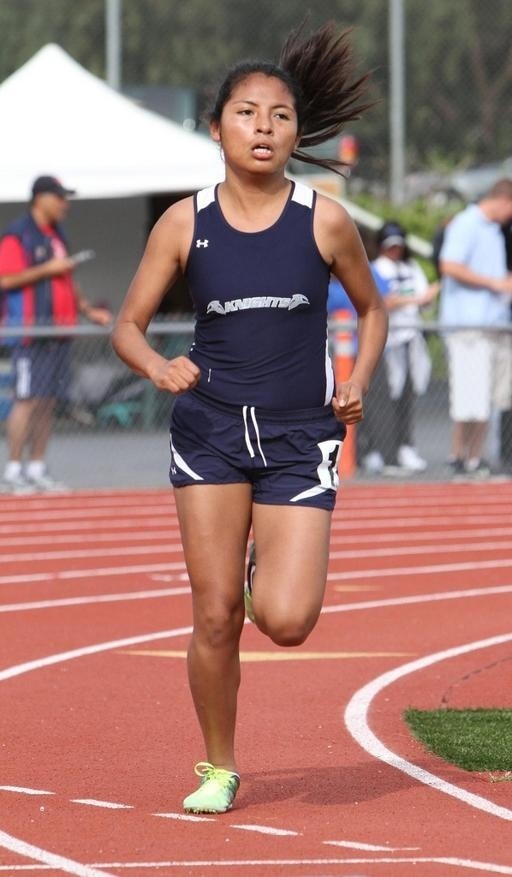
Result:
[379,222,414,250]
[31,175,77,198]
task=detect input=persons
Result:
[0,175,111,497]
[112,9,388,813]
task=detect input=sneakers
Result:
[243,541,259,625]
[180,769,243,817]
[0,460,67,498]
[361,442,510,486]
